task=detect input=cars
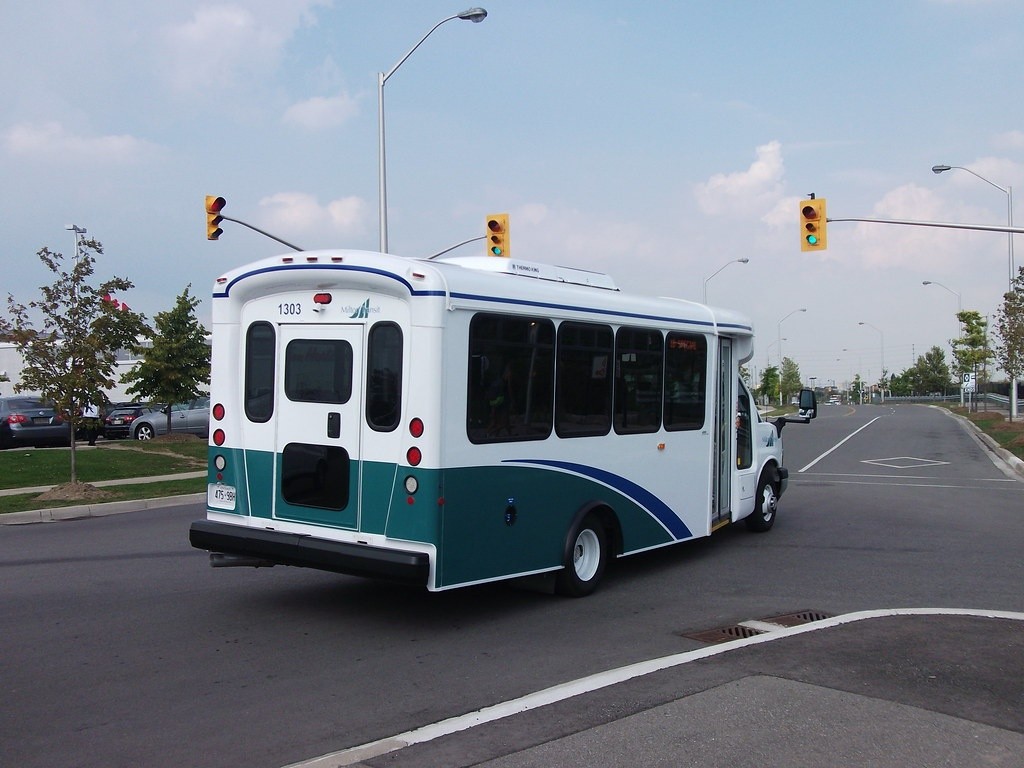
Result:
[98,401,165,440]
[128,395,210,441]
[0,394,71,449]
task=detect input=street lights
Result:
[63,222,87,306]
[930,164,1018,423]
[836,358,850,402]
[778,307,807,407]
[842,348,872,404]
[767,337,787,367]
[378,7,489,253]
[858,321,885,403]
[922,281,965,408]
[702,256,751,306]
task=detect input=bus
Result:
[189,256,817,600]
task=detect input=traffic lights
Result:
[799,198,828,253]
[204,194,228,241]
[486,211,510,258]
